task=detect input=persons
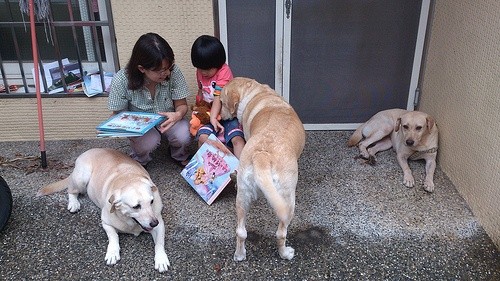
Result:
[109,32,193,167]
[190,34,245,190]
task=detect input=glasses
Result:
[160,63,175,72]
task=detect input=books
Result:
[95,110,167,139]
[180,132,241,206]
[82,74,114,97]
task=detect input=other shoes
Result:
[171,158,192,168]
[230,169,238,190]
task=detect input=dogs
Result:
[348,109,438,193]
[220,77,306,262]
[37,147,171,274]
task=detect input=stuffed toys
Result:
[188,100,223,135]
